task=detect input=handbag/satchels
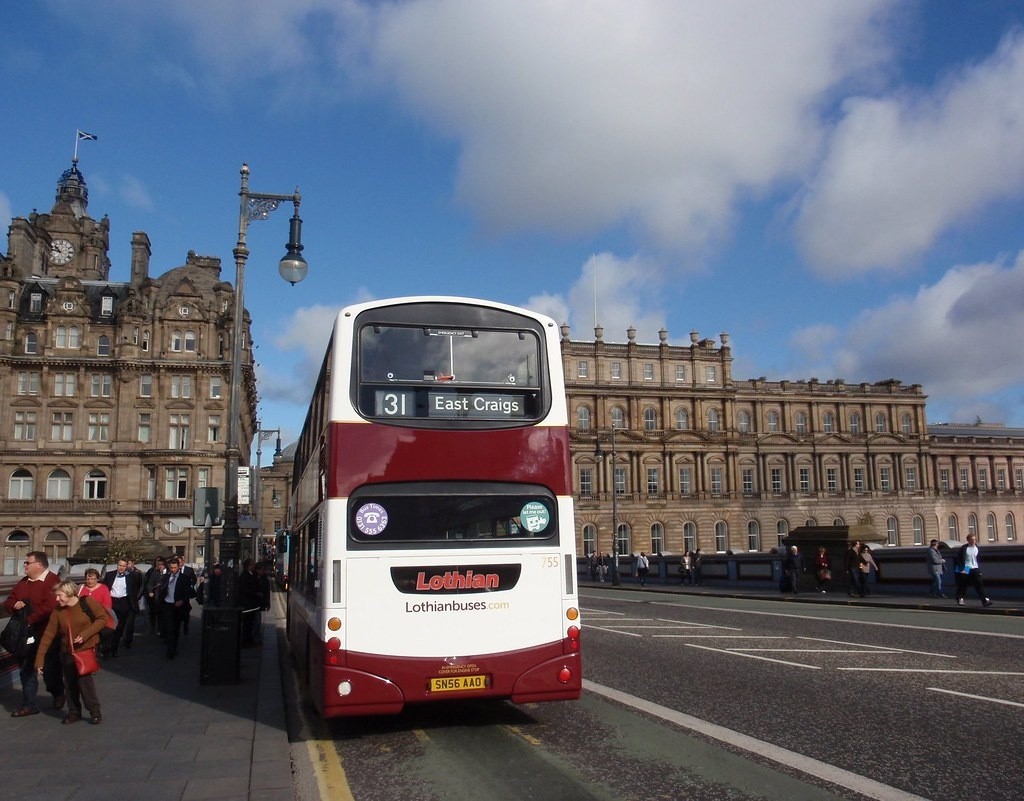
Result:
[820,567,831,580]
[646,567,649,573]
[0,607,26,653]
[679,567,685,572]
[73,648,99,675]
[633,571,639,578]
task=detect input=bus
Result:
[272,296,584,715]
[274,531,290,590]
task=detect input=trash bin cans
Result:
[201,605,244,686]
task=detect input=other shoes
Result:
[13,706,38,717]
[91,710,102,723]
[957,597,966,606]
[822,590,827,594]
[126,641,132,649]
[849,593,859,597]
[982,597,993,607]
[166,650,175,659]
[816,587,820,591]
[103,649,109,657]
[63,712,82,724]
[112,651,118,657]
[859,592,865,598]
[53,695,65,708]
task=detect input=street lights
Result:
[219,183,308,602]
[252,427,285,540]
[593,431,621,586]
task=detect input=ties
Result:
[179,568,182,574]
[161,574,177,590]
[117,567,133,578]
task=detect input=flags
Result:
[78,131,97,141]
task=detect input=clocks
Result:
[47,238,75,266]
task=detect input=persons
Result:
[78,555,143,676]
[679,548,706,587]
[4,551,67,717]
[143,555,197,657]
[955,534,993,608]
[636,552,649,587]
[815,546,831,594]
[35,580,108,724]
[588,551,608,583]
[196,554,271,613]
[258,541,275,576]
[845,541,879,597]
[926,539,949,599]
[784,546,806,594]
